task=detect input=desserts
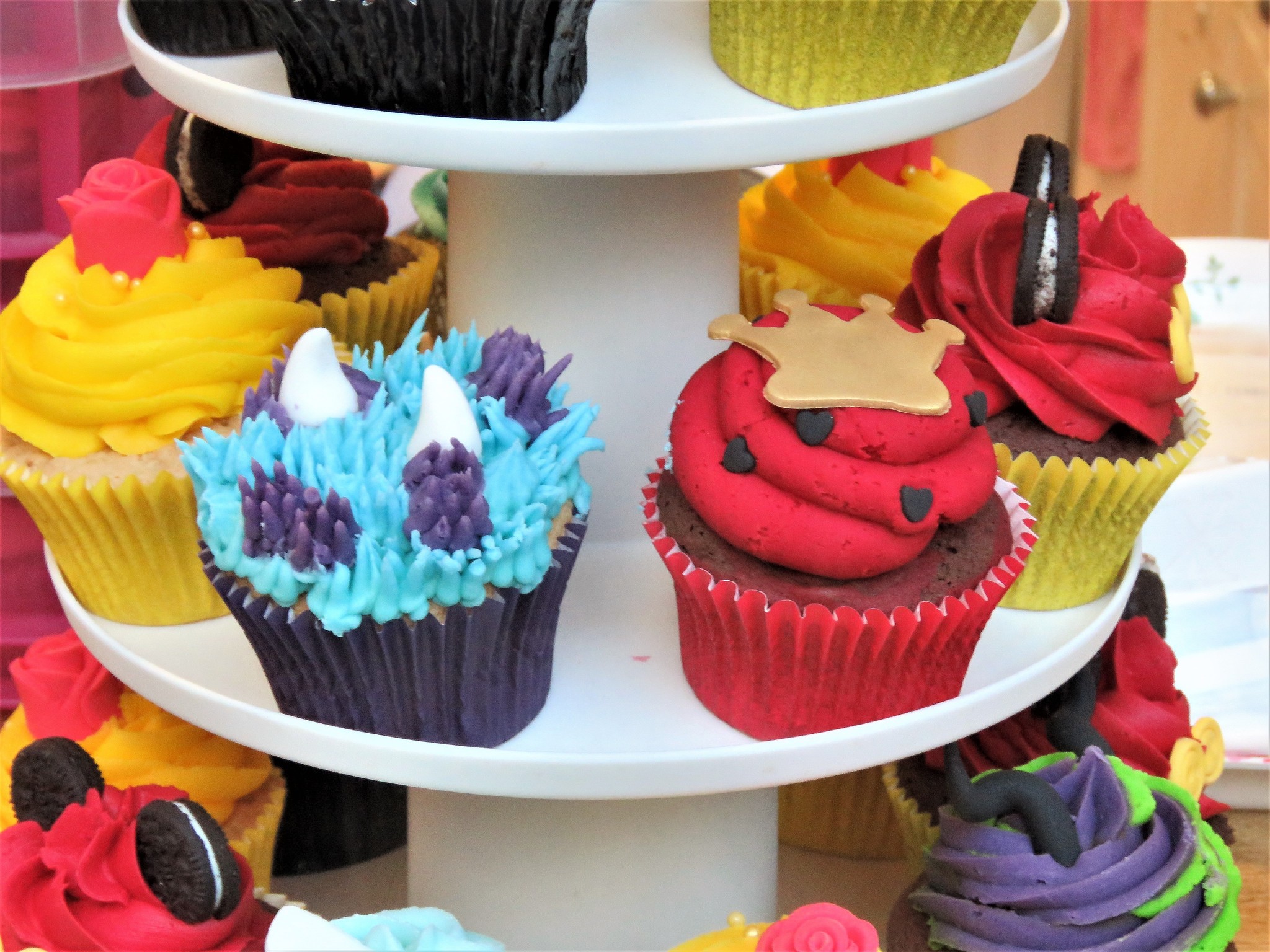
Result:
[0,110,1270,952]
[122,0,1033,133]
